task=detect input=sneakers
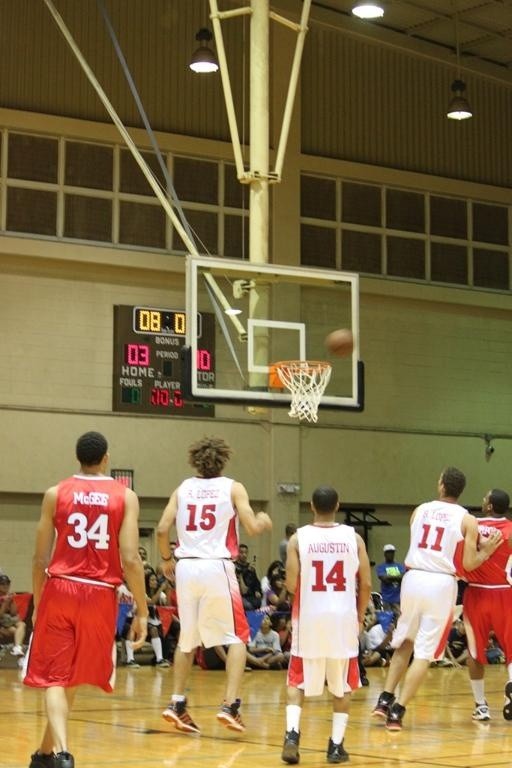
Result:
[28,749,55,768]
[385,703,405,730]
[124,658,140,667]
[326,737,348,762]
[55,752,75,768]
[371,691,395,720]
[163,698,201,732]
[9,646,24,656]
[216,699,247,731]
[282,728,300,764]
[155,658,170,667]
[471,699,490,720]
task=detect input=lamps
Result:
[351,0,385,20]
[189,27,219,74]
[445,15,474,121]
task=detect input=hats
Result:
[383,544,396,551]
[0,576,11,584]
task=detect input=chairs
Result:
[158,606,176,637]
[117,603,133,664]
[371,592,384,611]
[10,593,34,644]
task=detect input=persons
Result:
[462,489,512,720]
[2,574,34,657]
[114,542,181,670]
[194,543,294,671]
[373,467,505,730]
[376,543,405,616]
[155,434,274,733]
[281,487,373,764]
[22,431,150,768]
[357,592,505,669]
[282,523,297,564]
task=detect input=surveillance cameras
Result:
[487,446,494,454]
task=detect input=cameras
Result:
[278,566,285,578]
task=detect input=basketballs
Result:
[324,328,354,355]
